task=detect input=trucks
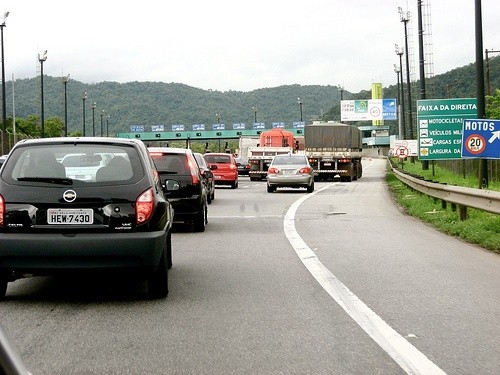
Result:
[233,127,305,181]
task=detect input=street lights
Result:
[393,63,401,141]
[0,10,11,156]
[100,110,104,137]
[91,101,96,137]
[62,73,70,137]
[398,6,415,140]
[106,115,110,137]
[81,91,88,137]
[394,43,406,140]
[37,53,47,138]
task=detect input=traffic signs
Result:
[460,119,500,160]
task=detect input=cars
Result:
[265,153,315,193]
[0,135,181,302]
[193,152,217,205]
[146,146,214,233]
[202,152,241,189]
[0,151,129,183]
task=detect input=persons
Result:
[23,150,132,182]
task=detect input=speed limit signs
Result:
[465,134,486,155]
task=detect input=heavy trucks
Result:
[303,120,363,182]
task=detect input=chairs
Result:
[23,151,65,178]
[95,156,132,181]
